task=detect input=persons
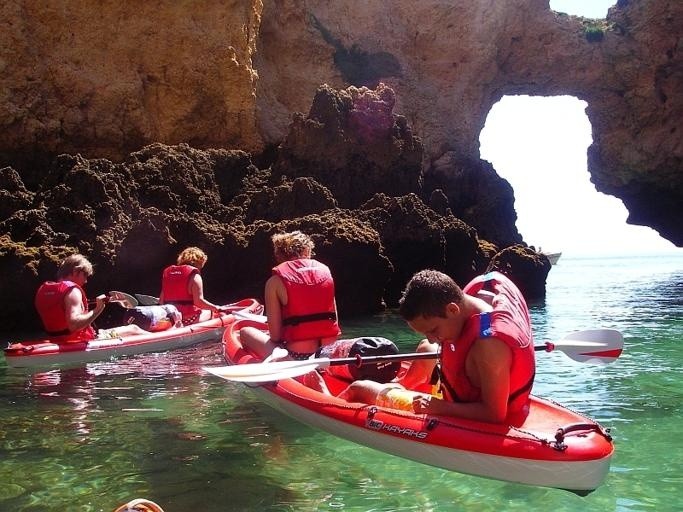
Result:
[33,253,108,342]
[238,230,342,362]
[158,246,223,325]
[303,268,535,428]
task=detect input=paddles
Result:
[88,291,138,309]
[201,329,623,383]
[135,294,267,323]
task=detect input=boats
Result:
[1,297,265,370]
[222,319,615,495]
